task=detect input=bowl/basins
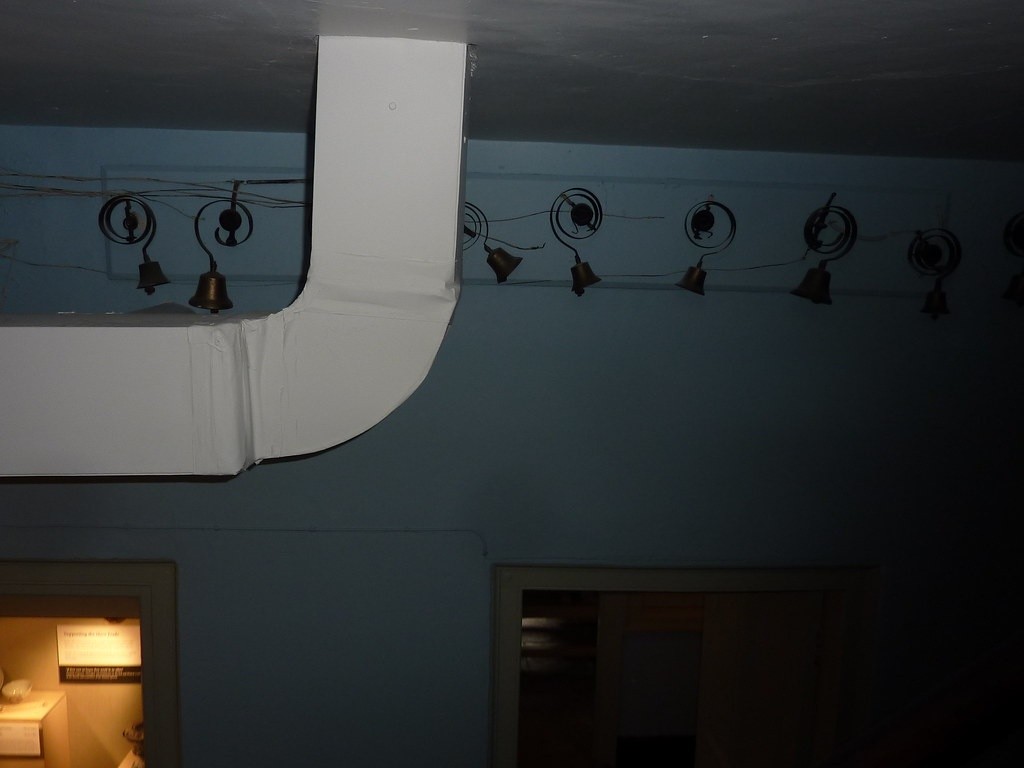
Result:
[2,679,32,702]
[0,669,4,690]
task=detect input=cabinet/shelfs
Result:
[0,687,71,768]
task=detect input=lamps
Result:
[453,185,963,320]
[98,182,253,317]
